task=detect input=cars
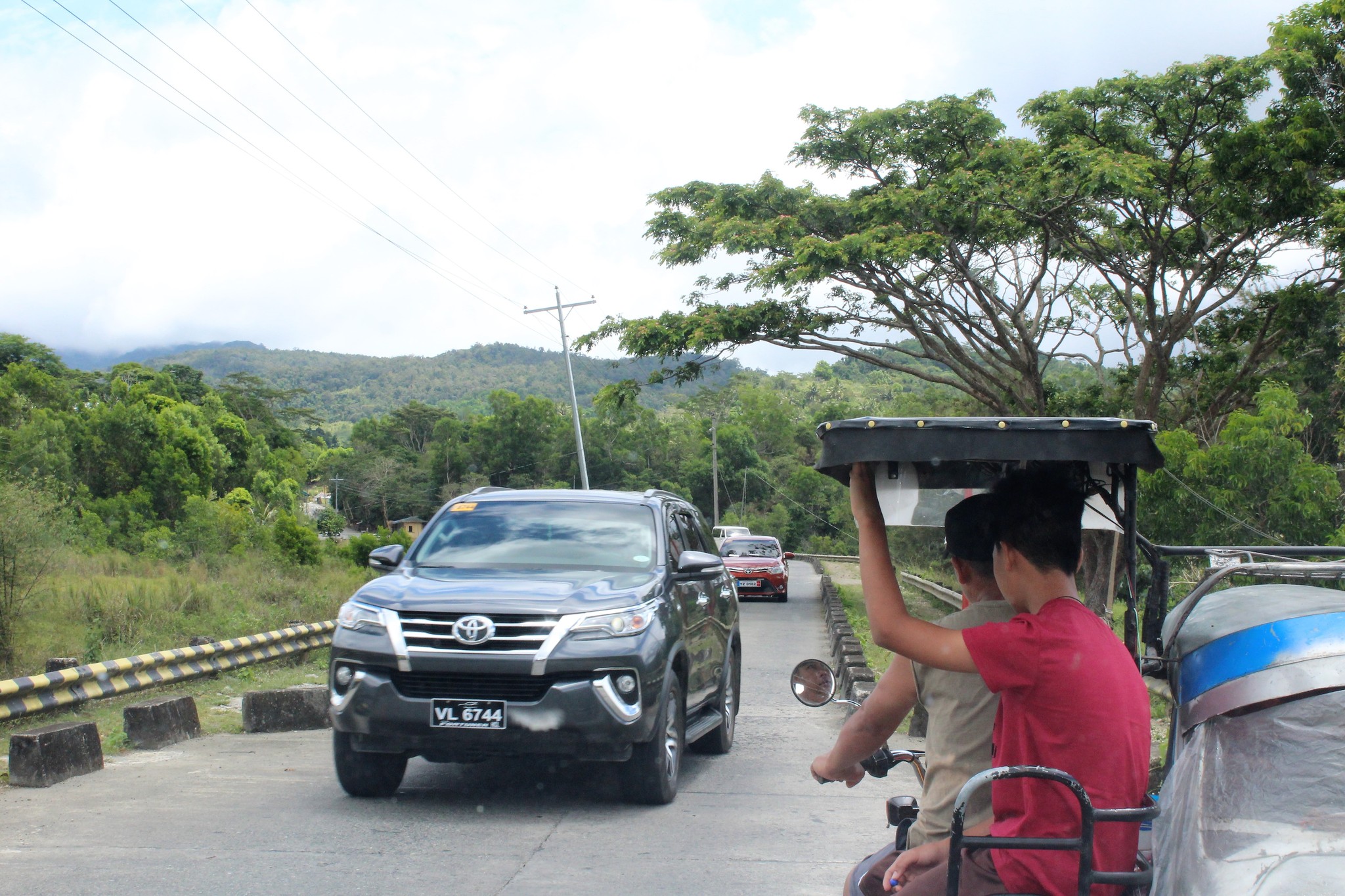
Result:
[718,535,795,602]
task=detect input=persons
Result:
[809,493,1026,896]
[847,461,1152,896]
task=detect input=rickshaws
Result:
[790,414,1344,896]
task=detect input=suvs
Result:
[712,526,754,553]
[327,486,743,806]
